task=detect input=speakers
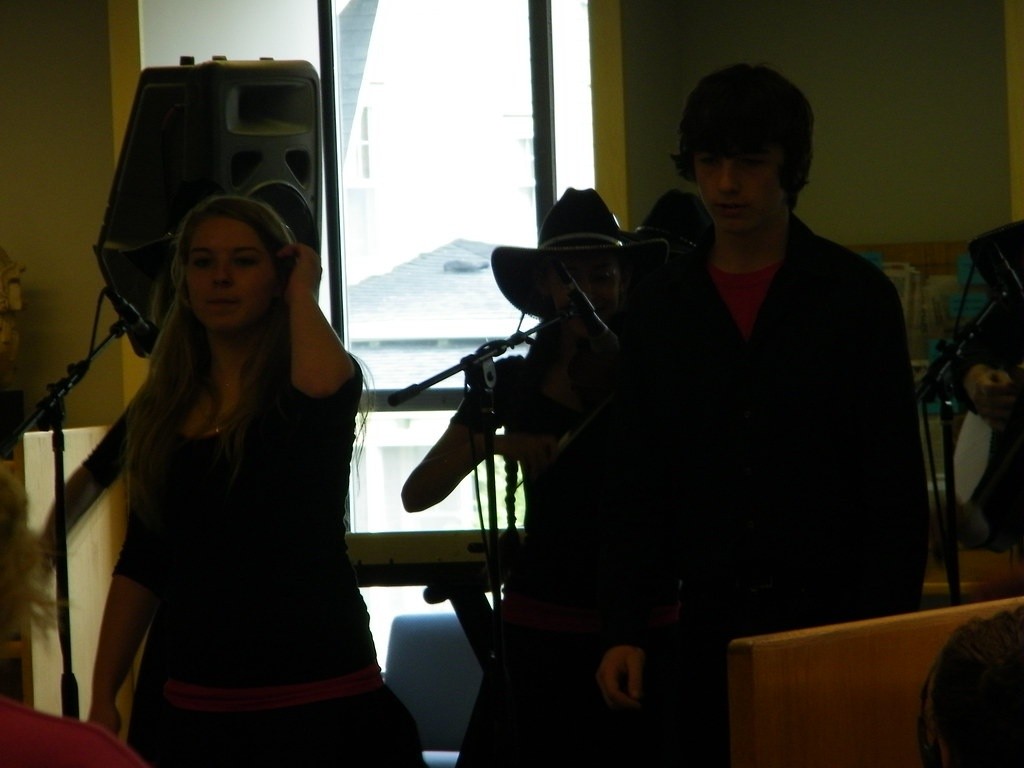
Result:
[92,56,324,358]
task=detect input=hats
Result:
[492,188,670,319]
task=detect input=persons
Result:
[85,190,430,767]
[405,63,1023,767]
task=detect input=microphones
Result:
[984,240,1024,318]
[554,261,620,360]
[104,286,160,354]
